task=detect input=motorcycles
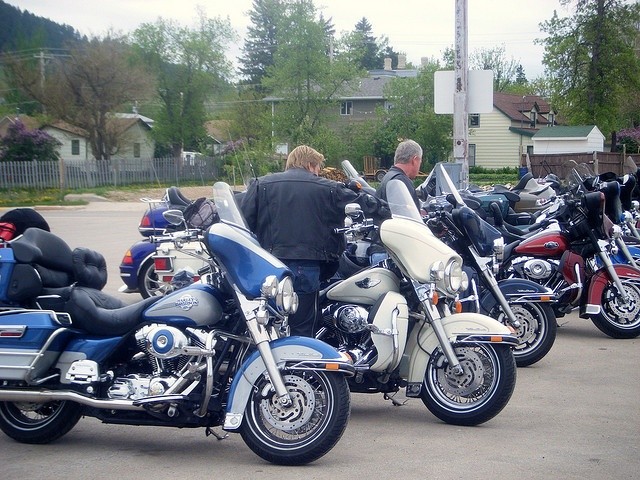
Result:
[422,163,640,271]
[121,160,376,299]
[119,180,518,427]
[426,163,559,368]
[422,168,640,335]
[422,160,640,243]
[422,155,640,298]
[0,181,355,466]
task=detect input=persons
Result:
[241,145,428,338]
[373,139,427,281]
